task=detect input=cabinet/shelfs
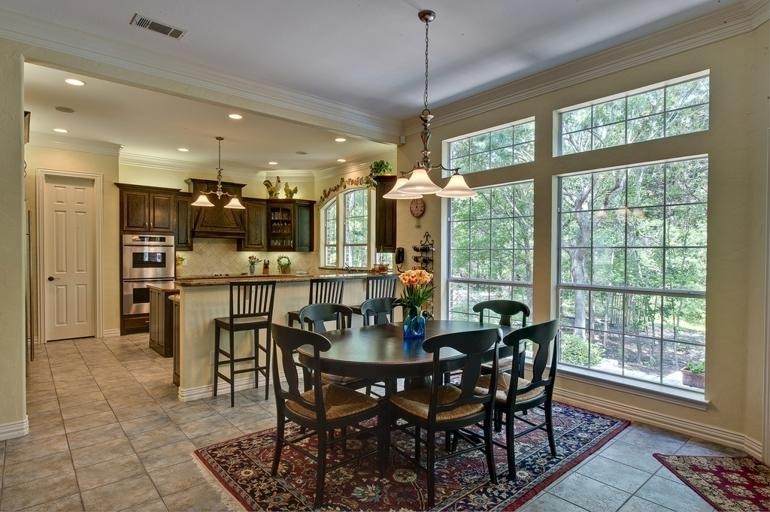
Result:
[148,287,173,358]
[373,174,396,253]
[114,182,317,251]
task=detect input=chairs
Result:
[299,303,372,434]
[385,327,503,507]
[361,297,399,326]
[473,299,531,415]
[288,278,345,392]
[350,276,398,326]
[270,323,390,508]
[453,318,561,479]
[214,281,276,407]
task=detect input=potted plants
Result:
[681,358,705,389]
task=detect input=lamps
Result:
[382,10,477,199]
[190,136,246,210]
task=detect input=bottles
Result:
[271,212,291,247]
[413,246,435,274]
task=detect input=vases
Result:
[402,307,426,340]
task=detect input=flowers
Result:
[390,269,437,307]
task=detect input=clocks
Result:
[409,199,426,218]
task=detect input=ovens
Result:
[122,234,175,315]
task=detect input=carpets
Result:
[191,399,631,512]
[652,452,770,512]
